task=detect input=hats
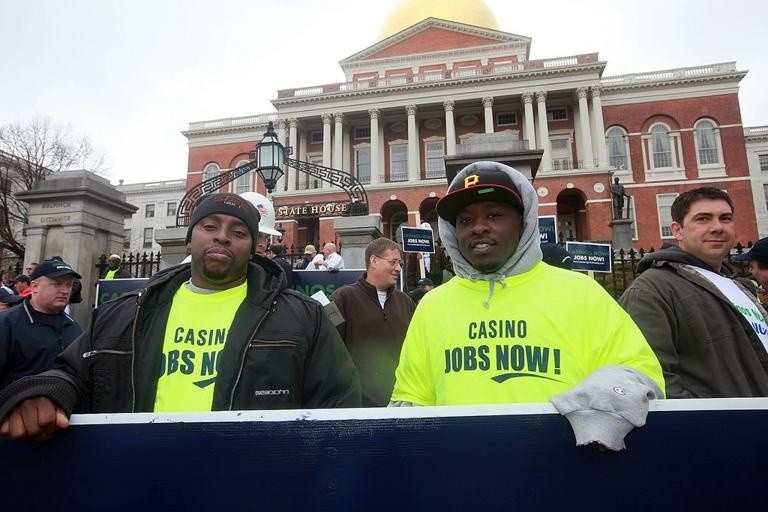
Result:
[109,255,121,262]
[185,192,262,252]
[435,170,522,221]
[10,274,29,283]
[304,244,317,254]
[0,285,25,304]
[733,236,767,264]
[31,255,82,279]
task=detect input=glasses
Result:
[377,255,405,266]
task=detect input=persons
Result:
[409,288,428,304]
[415,278,434,292]
[383,160,670,407]
[733,237,768,308]
[610,177,628,220]
[0,252,131,309]
[179,191,282,264]
[264,238,346,275]
[0,193,364,443]
[322,237,415,407]
[1,261,83,384]
[617,186,768,397]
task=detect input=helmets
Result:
[238,192,283,238]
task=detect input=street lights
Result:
[253,118,287,248]
